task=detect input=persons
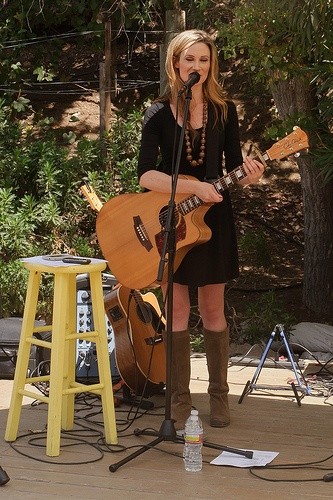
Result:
[138,29,264,442]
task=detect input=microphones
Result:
[178,72,200,97]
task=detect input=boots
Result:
[162,328,192,430]
[201,323,231,427]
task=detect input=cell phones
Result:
[62,257,91,265]
[42,254,72,260]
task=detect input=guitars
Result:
[93,126,310,291]
[80,183,168,396]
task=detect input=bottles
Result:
[183,410,204,472]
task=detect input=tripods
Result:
[109,91,254,472]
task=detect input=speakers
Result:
[0,317,45,380]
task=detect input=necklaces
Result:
[186,94,208,167]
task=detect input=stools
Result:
[4,262,118,457]
